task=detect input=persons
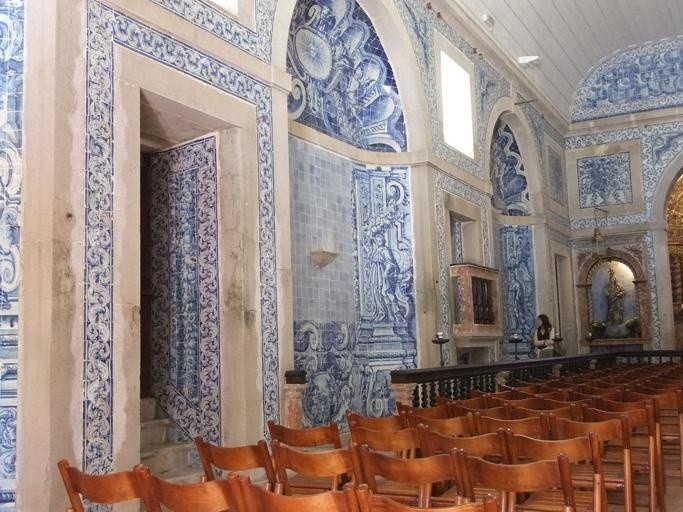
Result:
[532,313,555,358]
[602,269,627,323]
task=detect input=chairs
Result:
[57,363,682,511]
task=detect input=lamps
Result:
[591,208,607,261]
[312,248,338,270]
[432,332,452,366]
[509,333,524,361]
[552,333,563,357]
[586,332,594,355]
